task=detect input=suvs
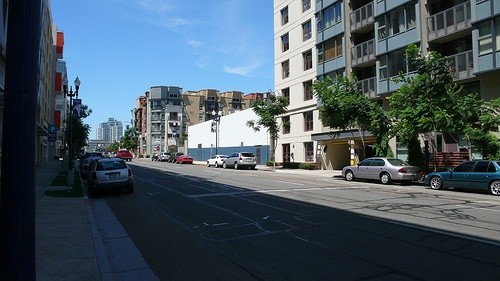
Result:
[222,152,256,170]
[76,152,134,196]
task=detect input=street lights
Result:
[211,109,222,155]
[62,75,81,171]
[171,121,181,152]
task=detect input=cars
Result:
[103,149,134,162]
[151,152,193,164]
[342,157,421,185]
[424,160,500,196]
[206,155,229,168]
